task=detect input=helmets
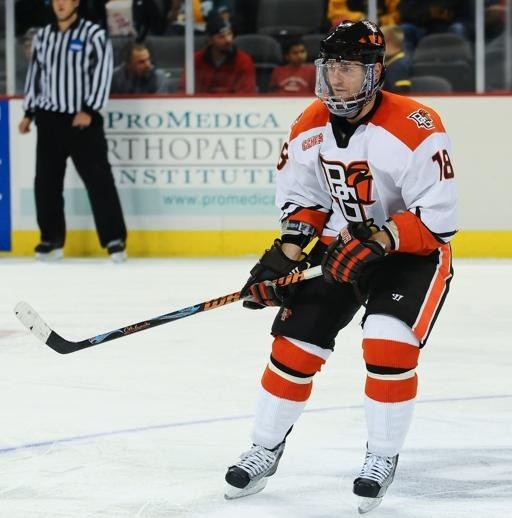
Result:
[314,20,388,117]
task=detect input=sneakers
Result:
[107,239,125,254]
[35,241,64,252]
[353,441,399,498]
[225,425,293,489]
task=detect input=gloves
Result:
[321,222,385,291]
[241,239,307,309]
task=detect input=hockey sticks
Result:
[12,263,324,354]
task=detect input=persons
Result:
[224,17,460,515]
[18,0,127,265]
[110,0,475,96]
